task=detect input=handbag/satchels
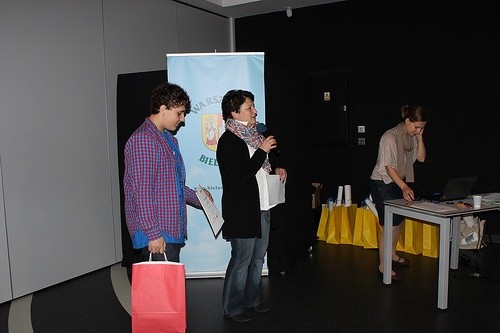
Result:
[130,249,188,333]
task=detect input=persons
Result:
[369,104,428,282]
[216,90,287,322]
[123,83,215,262]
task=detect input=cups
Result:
[473,195,482,208]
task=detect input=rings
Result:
[286,172,287,174]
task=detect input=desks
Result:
[382,192,500,312]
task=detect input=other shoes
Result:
[392,256,411,266]
[225,312,252,323]
[248,304,272,313]
[378,269,402,281]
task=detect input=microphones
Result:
[257,123,279,153]
